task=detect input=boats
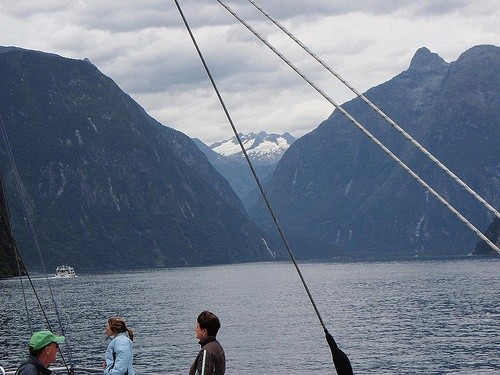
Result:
[55,265,76,278]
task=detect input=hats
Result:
[28,331,65,351]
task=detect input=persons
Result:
[14,330,65,375]
[189,310,225,375]
[103,317,135,375]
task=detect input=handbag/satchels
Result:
[107,363,128,375]
